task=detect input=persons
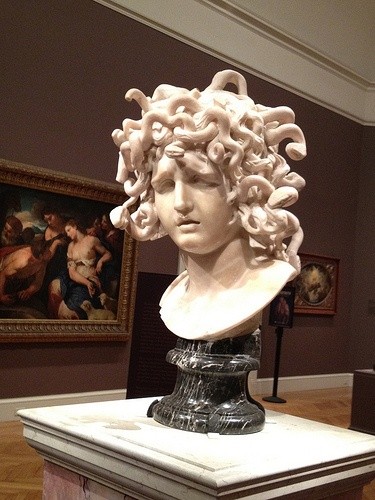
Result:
[106,68,308,345]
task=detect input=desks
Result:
[16,392,374,499]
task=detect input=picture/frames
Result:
[296,251,342,315]
[0,157,139,343]
